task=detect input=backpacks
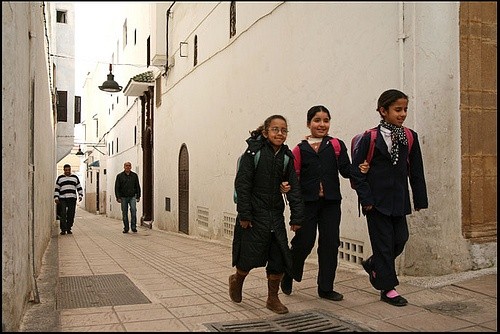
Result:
[351,128,414,191]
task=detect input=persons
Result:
[279,105,370,302]
[54,163,84,235]
[114,161,141,234]
[350,88,429,307]
[229,114,295,315]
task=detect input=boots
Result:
[266,278,289,314]
[229,272,247,302]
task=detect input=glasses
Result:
[266,128,290,135]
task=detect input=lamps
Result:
[75,145,84,155]
[98,64,122,92]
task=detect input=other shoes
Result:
[60,230,72,235]
[317,290,343,301]
[123,229,129,233]
[361,255,409,306]
[132,229,138,232]
[280,274,292,295]
